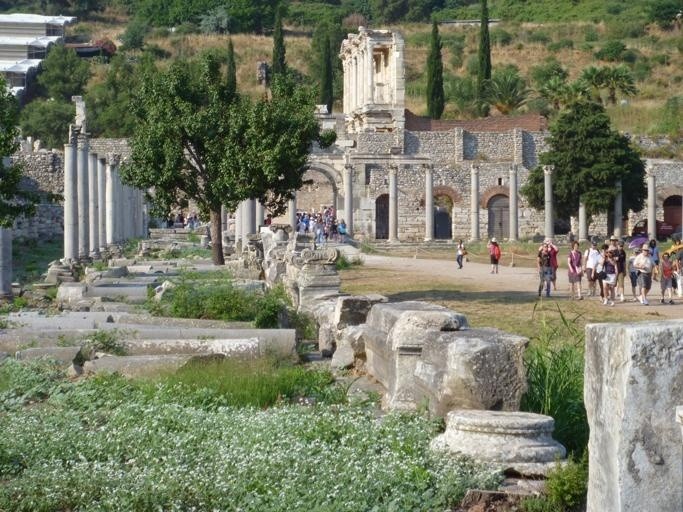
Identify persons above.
[264,214,272,225]
[487,238,500,273]
[296,204,347,243]
[567,235,683,306]
[537,237,559,297]
[456,239,464,269]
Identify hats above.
[591,236,652,250]
[491,237,496,242]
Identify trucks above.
[631,219,680,241]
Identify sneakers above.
[601,295,675,306]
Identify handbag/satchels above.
[575,266,583,276]
[597,272,606,278]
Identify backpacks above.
[494,245,501,260]
